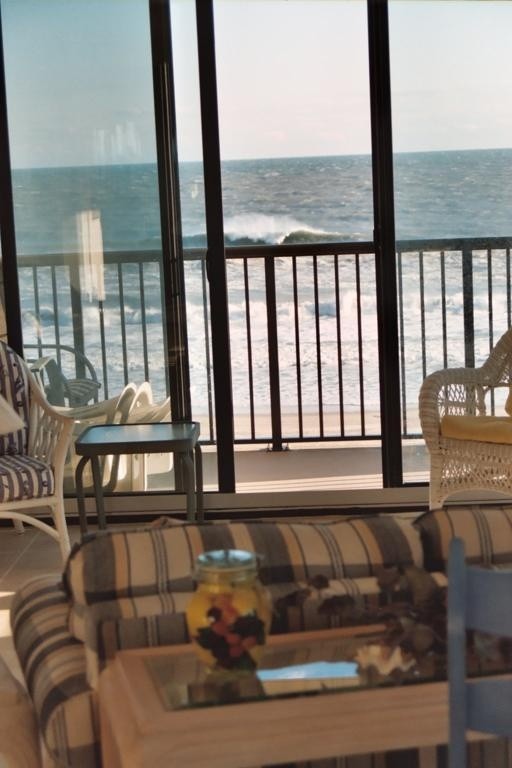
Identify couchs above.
[10,504,512,768]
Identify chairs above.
[419,329,512,512]
[0,341,76,563]
[23,344,101,408]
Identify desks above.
[75,420,202,536]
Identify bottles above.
[186,550,274,676]
